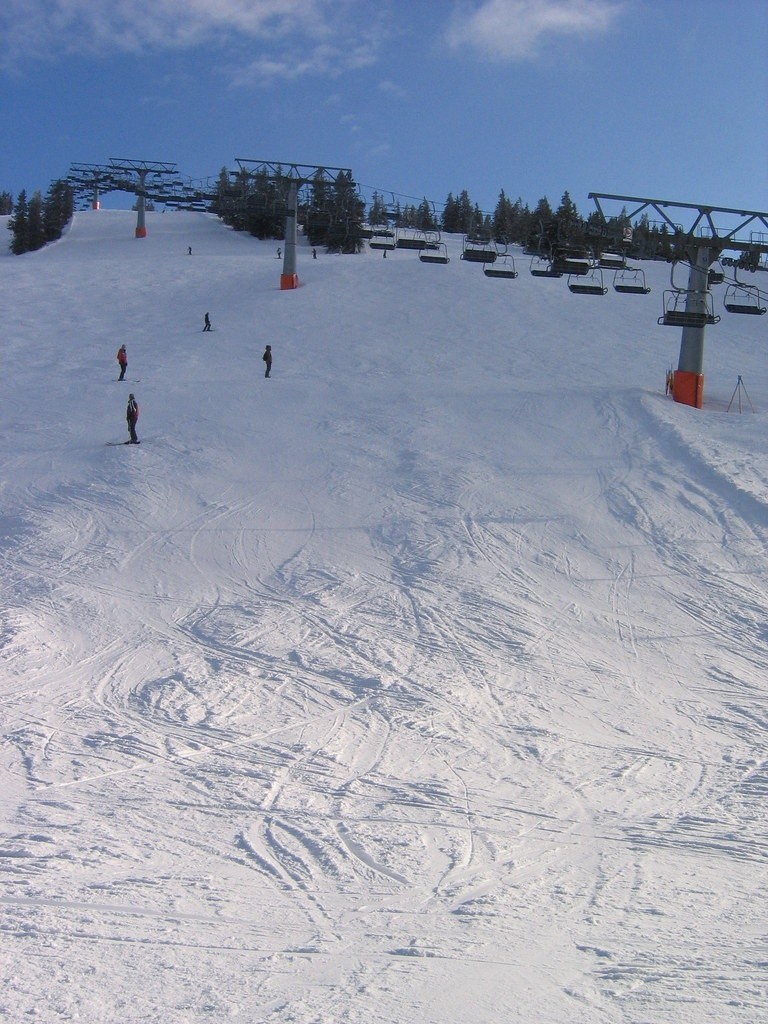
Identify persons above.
[126,394,139,444]
[117,345,128,381]
[188,247,192,255]
[202,312,211,332]
[312,248,317,259]
[265,345,272,378]
[707,269,715,289]
[277,248,281,258]
